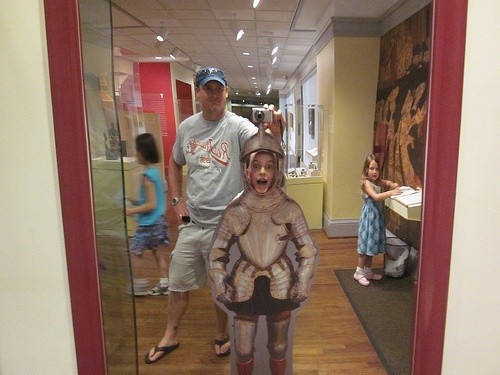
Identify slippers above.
[144,342,179,364]
[215,333,230,356]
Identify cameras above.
[252,107,272,123]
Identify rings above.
[179,213,182,216]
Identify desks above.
[384,186,422,221]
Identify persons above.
[144,67,286,367]
[123,132,172,295]
[353,154,403,286]
[246,150,275,194]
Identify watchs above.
[172,195,182,206]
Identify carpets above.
[334,268,414,375]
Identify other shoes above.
[365,272,382,281]
[353,272,369,286]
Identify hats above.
[196,68,227,88]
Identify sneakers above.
[147,284,169,296]
[126,286,152,295]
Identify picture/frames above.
[309,109,314,135]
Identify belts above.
[182,216,194,223]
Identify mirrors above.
[44,0,468,375]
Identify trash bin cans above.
[384,237,411,279]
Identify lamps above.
[268,39,278,55]
[267,51,277,64]
[156,27,169,41]
[233,14,244,40]
[170,48,179,59]
